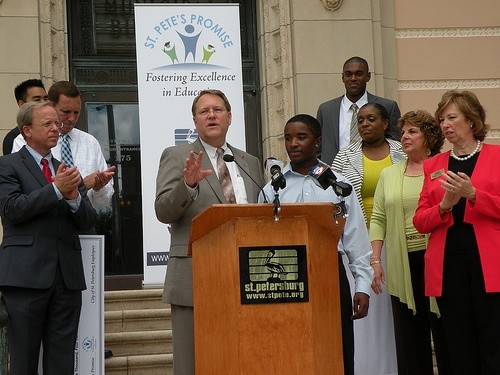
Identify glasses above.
[26,119,63,128]
[196,105,228,115]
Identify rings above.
[452,186,455,189]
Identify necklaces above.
[451,139,480,161]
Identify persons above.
[317,57,401,166]
[369,109,445,375]
[0,101,98,375]
[412,88,500,375]
[331,102,408,375]
[153,89,266,374]
[258,113,375,375]
[10,80,116,359]
[3,78,49,154]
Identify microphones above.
[309,163,352,197]
[223,154,267,203]
[270,165,286,207]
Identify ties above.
[216,147,236,204]
[41,159,53,185]
[60,133,75,168]
[350,104,361,145]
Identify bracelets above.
[370,256,381,260]
[370,261,381,266]
[439,202,453,212]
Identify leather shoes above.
[104,350,113,359]
